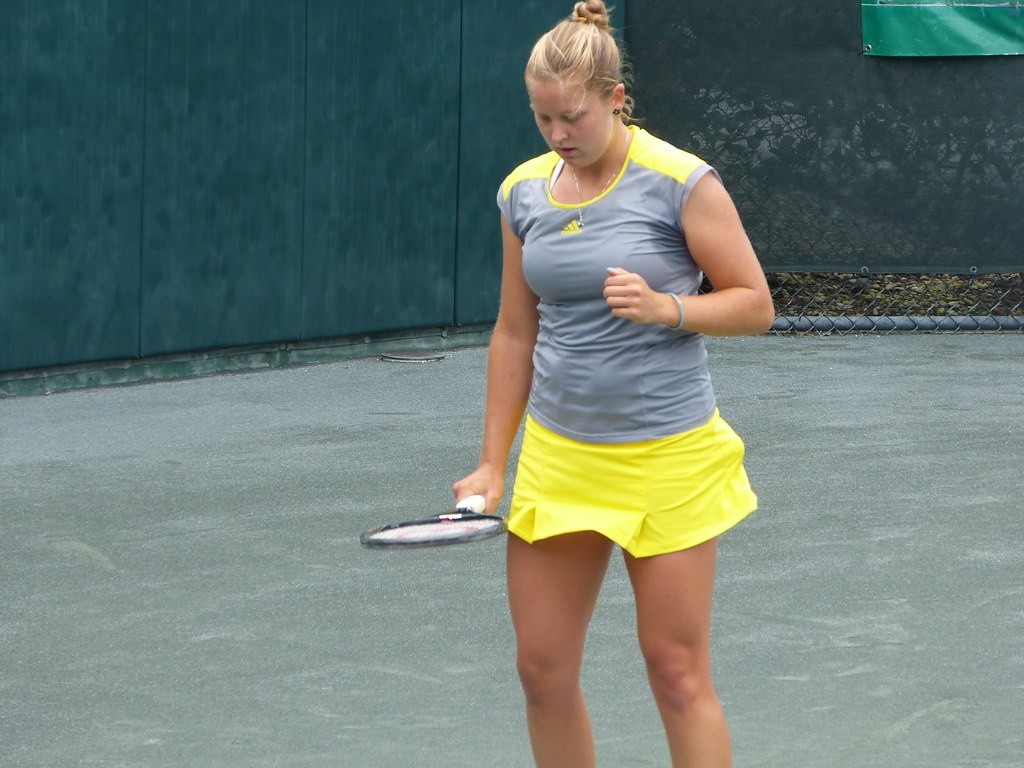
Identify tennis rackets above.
[359,494,508,550]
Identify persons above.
[452,0,775,768]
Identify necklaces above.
[569,126,635,228]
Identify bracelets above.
[665,291,685,334]
